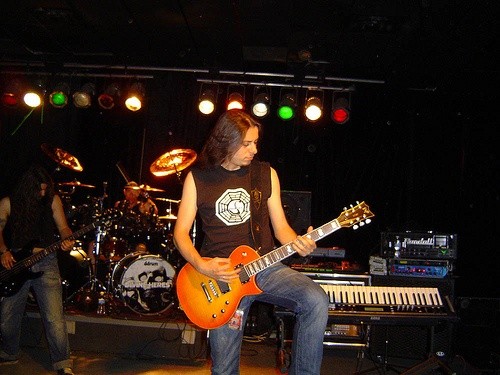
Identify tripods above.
[357,325,405,375]
[62,224,122,319]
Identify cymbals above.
[124,183,165,193]
[158,213,178,221]
[150,149,198,177]
[58,182,95,189]
[41,142,85,172]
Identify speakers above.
[369,275,458,362]
[280,190,312,235]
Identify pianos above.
[272,284,457,375]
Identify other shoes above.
[57,367,74,375]
[0,358,19,365]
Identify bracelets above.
[0,249,9,256]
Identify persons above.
[0,165,158,375]
[172,107,329,375]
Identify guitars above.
[0,209,125,297]
[174,201,375,332]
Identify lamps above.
[123,83,146,113]
[72,82,96,108]
[224,84,246,111]
[277,88,298,121]
[98,82,121,110]
[0,77,25,109]
[49,82,72,108]
[197,82,220,117]
[304,90,325,122]
[21,80,50,108]
[331,90,351,125]
[250,86,273,120]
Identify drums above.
[70,200,164,245]
[111,250,177,315]
